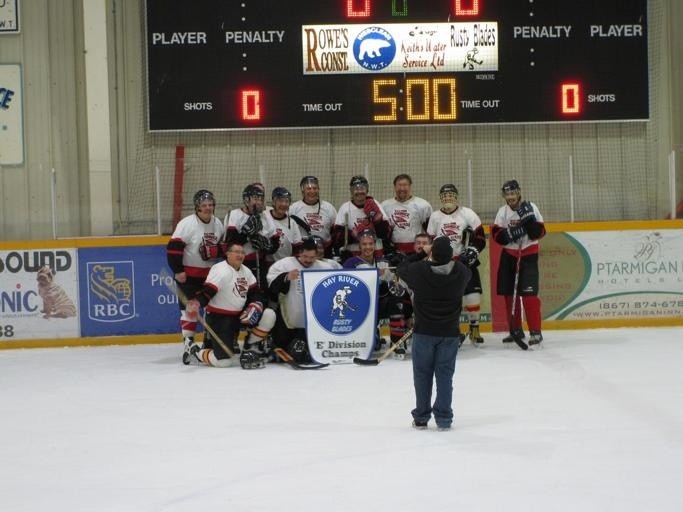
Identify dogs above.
[36,266,77,319]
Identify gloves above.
[517,200,536,232]
[240,301,264,327]
[185,299,200,320]
[504,223,525,243]
[460,248,479,269]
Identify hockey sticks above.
[509,238,528,350]
[266,338,330,370]
[156,269,240,364]
[291,214,313,240]
[353,326,412,366]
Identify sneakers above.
[230,340,407,369]
[528,334,543,345]
[501,330,523,342]
[468,325,484,343]
[437,421,450,430]
[183,342,199,364]
[411,421,427,430]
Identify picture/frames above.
[0,0,21,35]
[0,62,24,168]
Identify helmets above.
[349,177,366,186]
[503,180,519,192]
[193,189,214,204]
[243,183,263,196]
[300,176,317,189]
[440,184,457,204]
[272,188,289,199]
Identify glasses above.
[226,250,245,254]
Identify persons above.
[400,235,472,430]
[166,173,487,370]
[491,180,546,346]
